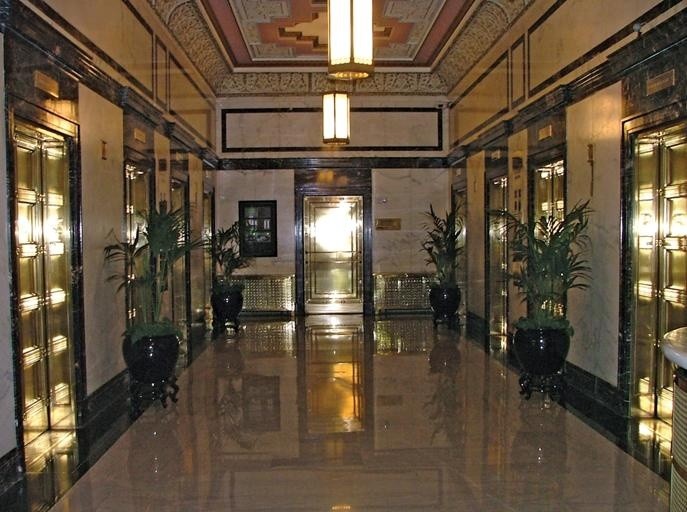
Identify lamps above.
[322,94,351,145]
[327,0,373,81]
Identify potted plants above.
[418,200,468,328]
[488,198,595,400]
[103,207,208,409]
[203,221,257,333]
